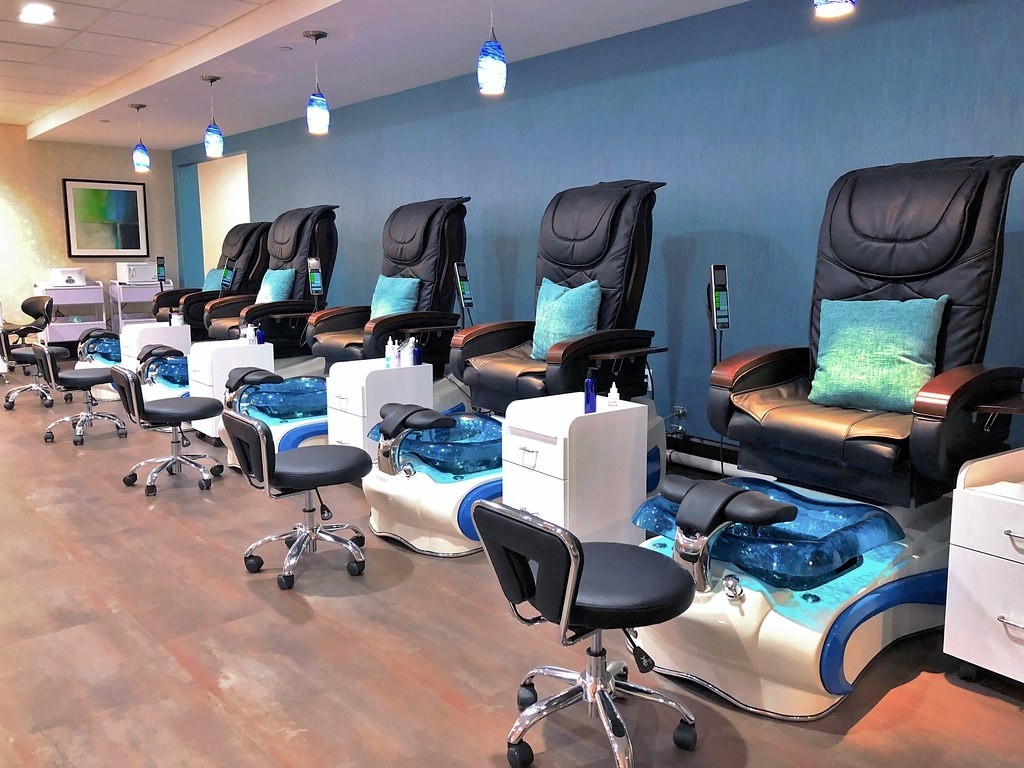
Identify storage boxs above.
[47,267,88,287]
[117,261,159,284]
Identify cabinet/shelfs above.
[502,391,647,546]
[33,280,107,347]
[326,357,433,464]
[108,278,174,334]
[188,339,276,447]
[943,446,1024,684]
[119,322,192,373]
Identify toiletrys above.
[583,366,600,414]
[167,305,185,326]
[607,382,621,409]
[385,336,422,369]
[238,321,265,346]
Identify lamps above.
[200,75,224,158]
[302,30,330,135]
[476,0,507,97]
[813,0,857,18]
[128,103,150,173]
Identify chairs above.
[0,154,1024,768]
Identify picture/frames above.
[61,178,150,258]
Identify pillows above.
[255,267,296,305]
[202,267,233,291]
[530,276,601,361]
[807,293,951,414]
[370,273,421,321]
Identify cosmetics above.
[168,306,184,326]
[607,381,619,410]
[239,322,264,345]
[384,336,421,368]
[584,367,600,414]
